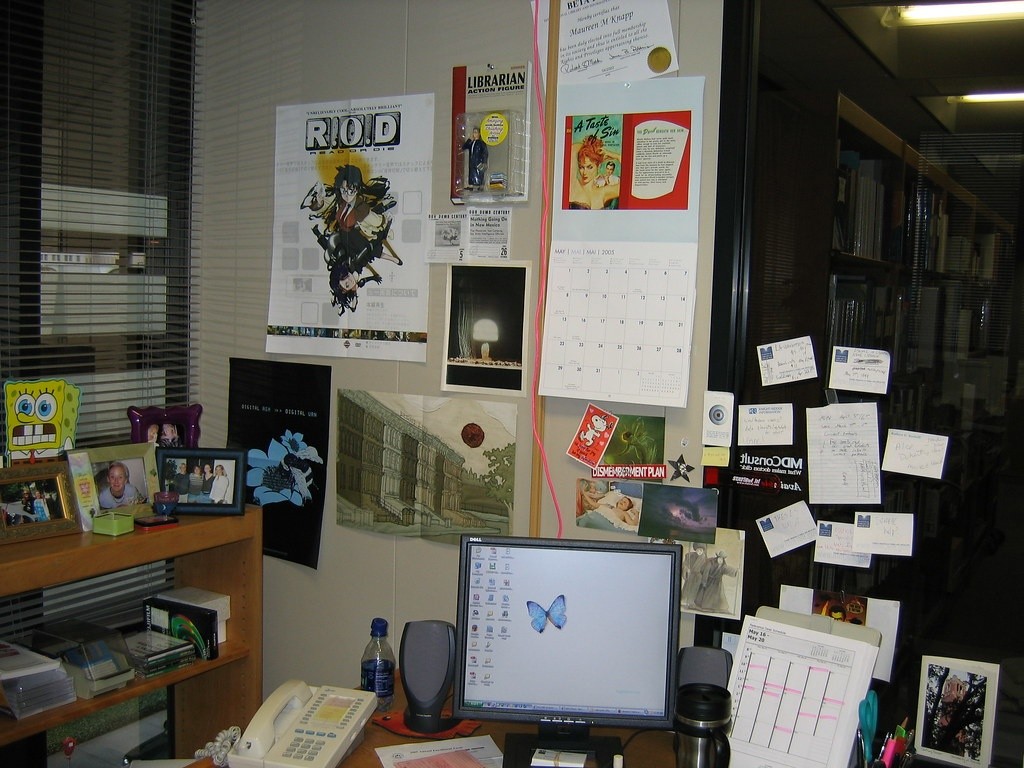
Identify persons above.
[148,424,182,448]
[462,127,489,187]
[21,490,62,523]
[99,462,142,509]
[173,463,229,503]
[579,488,639,527]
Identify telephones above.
[225,679,379,768]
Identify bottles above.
[360,618,396,710]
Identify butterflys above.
[525,594,567,635]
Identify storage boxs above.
[92,512,135,537]
[142,586,230,662]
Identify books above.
[0,640,60,680]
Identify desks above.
[184,664,678,768]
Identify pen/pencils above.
[856,717,917,768]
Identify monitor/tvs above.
[451,532,683,768]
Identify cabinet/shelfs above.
[1,501,264,760]
[830,90,1016,682]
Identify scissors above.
[859,691,878,768]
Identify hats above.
[693,542,706,551]
[715,551,728,559]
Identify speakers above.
[676,646,733,688]
[398,619,457,734]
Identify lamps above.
[473,319,499,362]
[879,0,1024,30]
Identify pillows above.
[598,492,642,512]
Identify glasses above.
[23,493,29,494]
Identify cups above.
[673,683,733,768]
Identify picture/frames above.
[127,403,203,449]
[155,447,248,516]
[66,443,160,532]
[0,461,83,548]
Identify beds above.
[576,503,641,535]
